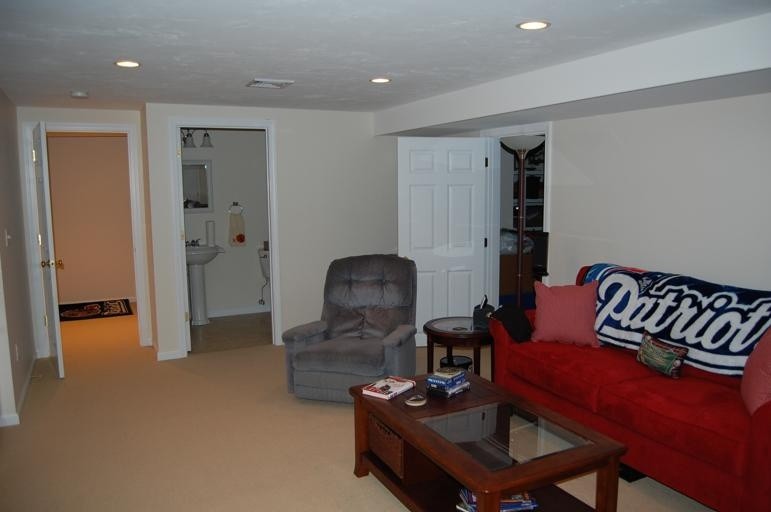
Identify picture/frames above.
[182,159,214,213]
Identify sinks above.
[186,244,224,264]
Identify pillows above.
[741,326,771,416]
[532,281,600,348]
[635,330,688,379]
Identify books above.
[426,381,473,399]
[361,375,417,399]
[428,378,467,391]
[426,367,467,387]
[456,484,540,512]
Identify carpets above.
[59,299,133,322]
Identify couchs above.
[488,263,771,512]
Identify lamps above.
[181,129,213,148]
[501,136,545,310]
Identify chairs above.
[282,254,417,404]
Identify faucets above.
[191,240,195,245]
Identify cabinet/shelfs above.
[501,147,544,232]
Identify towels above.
[230,213,246,247]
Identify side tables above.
[423,317,492,376]
